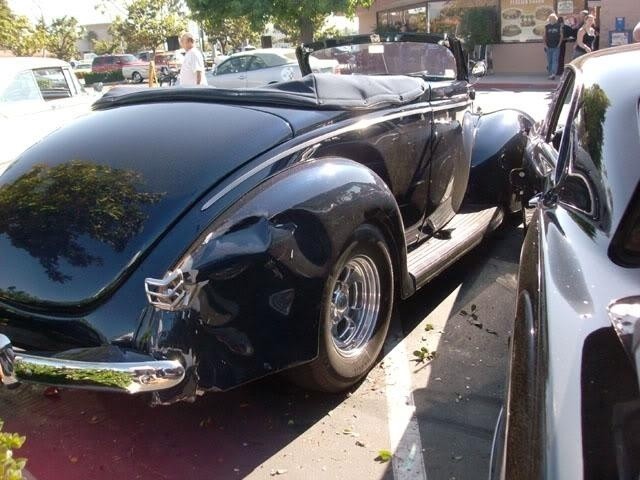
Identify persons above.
[542,9,597,80]
[179,32,208,86]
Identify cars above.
[67,49,189,85]
[203,47,341,89]
[0,28,543,412]
[0,56,107,178]
[484,35,640,480]
